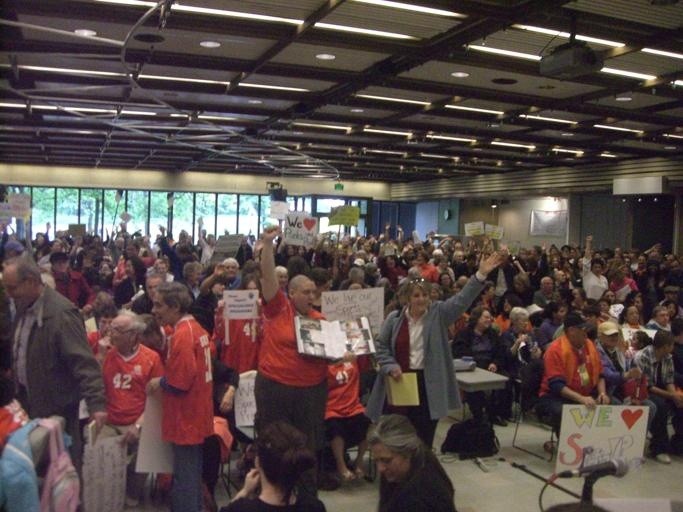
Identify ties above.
[11,316,25,393]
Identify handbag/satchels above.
[440,416,502,461]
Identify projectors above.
[539,47,604,80]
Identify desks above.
[447,366,509,423]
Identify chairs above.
[510,358,557,463]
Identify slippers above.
[335,469,358,483]
[368,470,376,481]
[349,462,364,480]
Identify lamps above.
[490,199,497,209]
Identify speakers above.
[272,189,287,202]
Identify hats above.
[354,258,366,266]
[49,252,69,263]
[664,286,681,293]
[598,321,618,336]
[526,304,545,318]
[5,240,25,253]
[564,310,594,328]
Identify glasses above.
[408,278,425,285]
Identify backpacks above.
[37,417,81,512]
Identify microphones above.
[557,457,628,478]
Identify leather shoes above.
[489,414,507,427]
[656,453,672,464]
